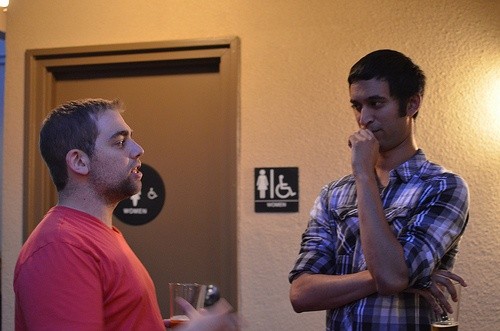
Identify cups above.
[169,282,207,320]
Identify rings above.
[424,281,434,289]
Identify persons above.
[10,97,233,331]
[287,47,471,330]
